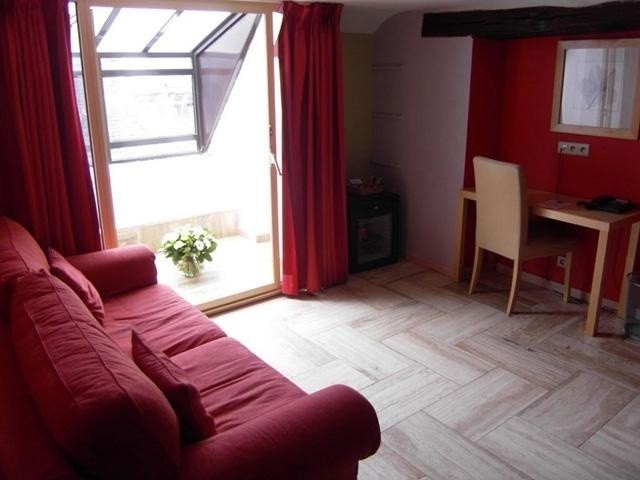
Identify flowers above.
[157,223,217,271]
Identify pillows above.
[127,327,217,445]
[46,248,105,326]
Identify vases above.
[177,259,206,278]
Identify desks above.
[451,188,640,338]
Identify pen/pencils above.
[362,175,384,185]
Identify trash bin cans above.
[625,271,640,342]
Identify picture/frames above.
[548,38,640,141]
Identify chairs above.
[468,155,583,317]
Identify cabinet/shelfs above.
[345,188,407,276]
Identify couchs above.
[1,215,380,480]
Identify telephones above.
[589,195,637,213]
